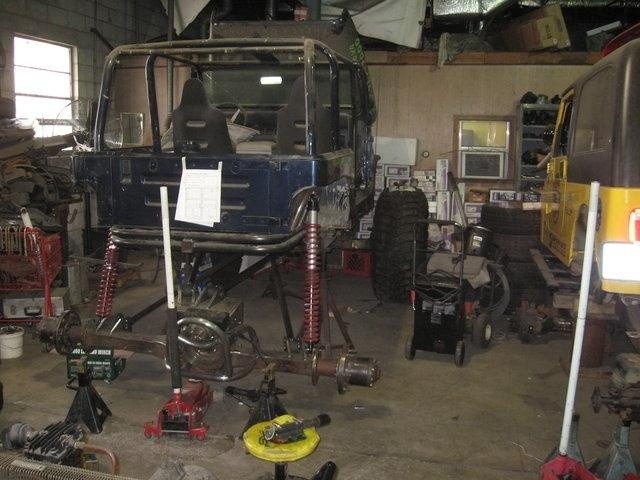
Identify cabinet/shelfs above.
[514,102,560,192]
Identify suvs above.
[35,31,429,396]
[522,33,640,331]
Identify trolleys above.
[406,212,499,368]
[0,222,65,356]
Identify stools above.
[243,414,321,480]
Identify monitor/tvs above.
[458,121,511,151]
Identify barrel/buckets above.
[0,326,25,359]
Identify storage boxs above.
[358,158,542,241]
[491,5,574,52]
